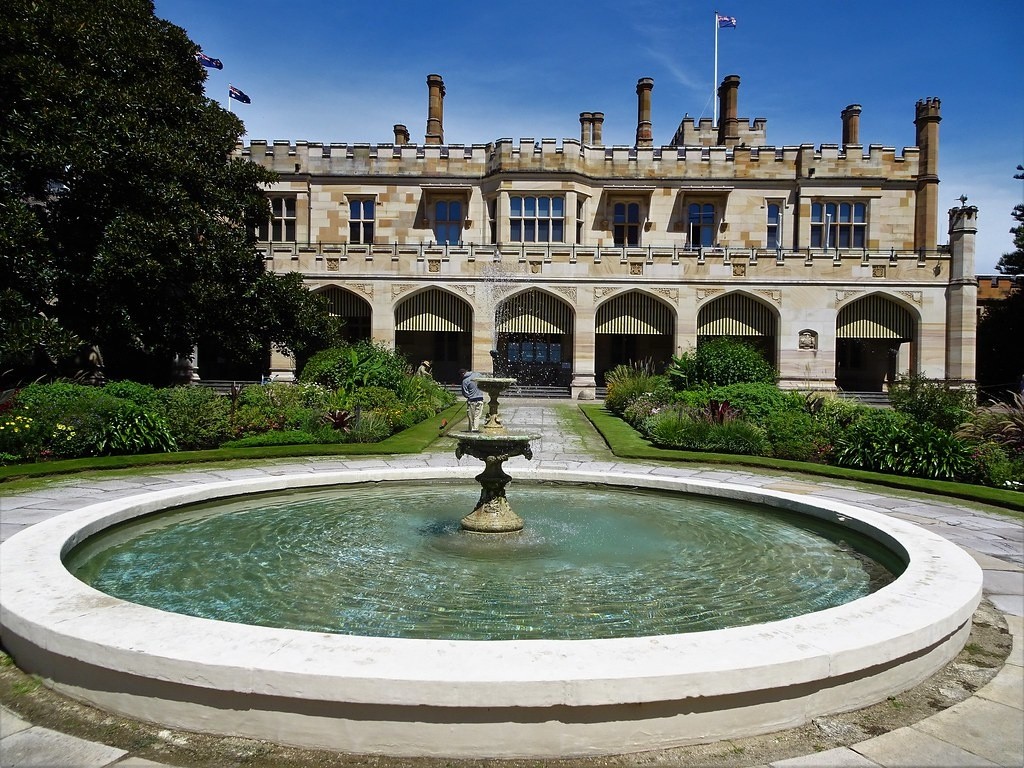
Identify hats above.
[421,361,430,367]
[458,369,466,380]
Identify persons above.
[416,361,431,379]
[459,368,485,432]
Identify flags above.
[718,15,737,30]
